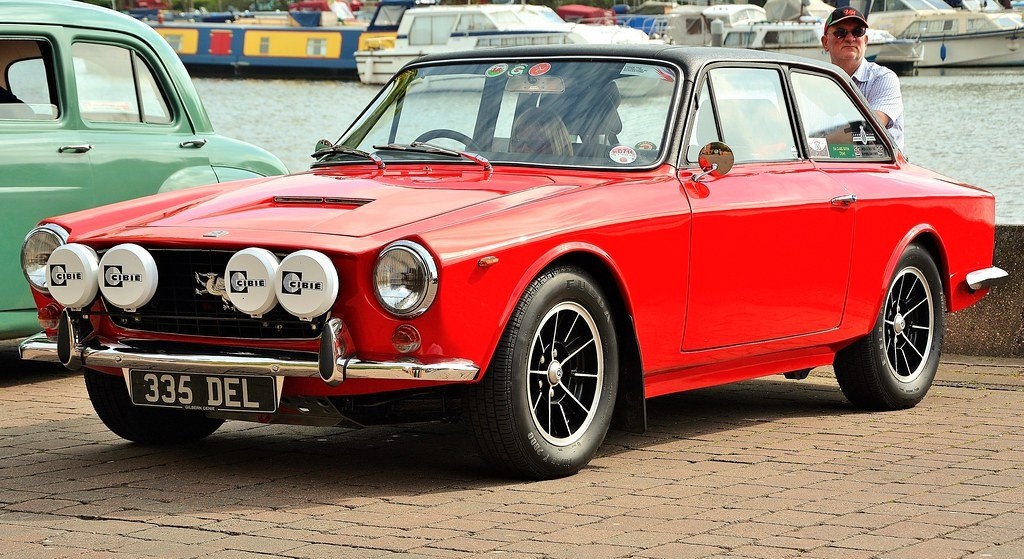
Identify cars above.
[0,0,291,351]
[15,46,1011,477]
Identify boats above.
[353,0,1024,98]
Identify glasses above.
[828,26,867,39]
[510,138,551,153]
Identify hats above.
[824,6,869,34]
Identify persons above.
[509,107,574,156]
[808,7,904,158]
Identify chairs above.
[559,88,622,157]
[689,96,789,161]
